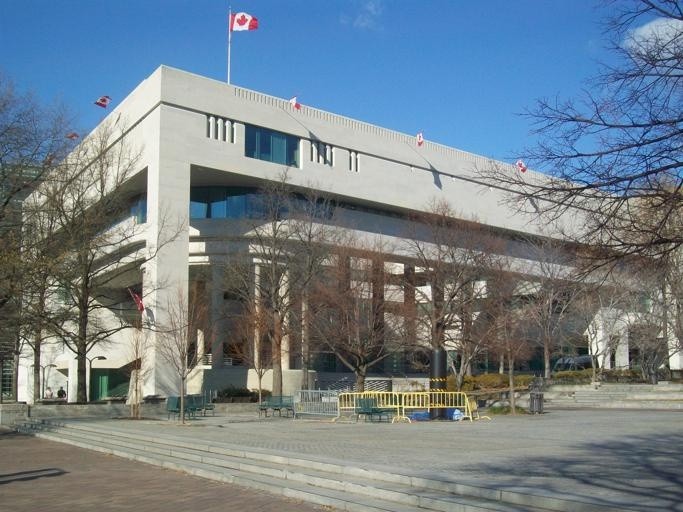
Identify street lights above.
[31,364,57,399]
[75,355,106,402]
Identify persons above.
[57,387,66,398]
[44,387,53,399]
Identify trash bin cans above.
[530,393,543,414]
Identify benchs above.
[167,394,215,421]
[353,398,396,423]
[256,395,294,418]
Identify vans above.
[552,356,599,372]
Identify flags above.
[128,288,144,313]
[64,133,80,139]
[516,160,527,173]
[416,131,424,146]
[230,12,258,31]
[290,97,301,109]
[95,96,112,108]
